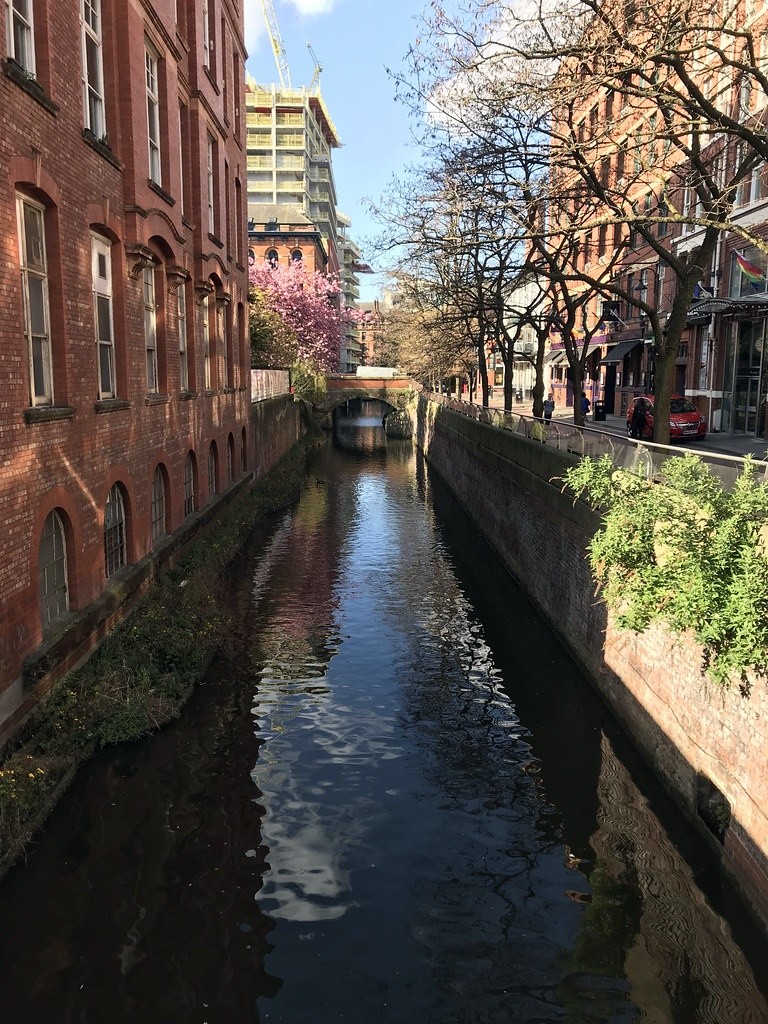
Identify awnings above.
[549,346,600,370]
[597,339,643,365]
[542,349,563,364]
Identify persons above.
[632,396,647,438]
[543,392,554,426]
[580,393,591,415]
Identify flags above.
[733,250,766,291]
[693,284,712,301]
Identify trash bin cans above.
[515,390,523,404]
[488,385,491,395]
[592,400,607,421]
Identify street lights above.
[633,265,660,396]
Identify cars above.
[625,393,707,444]
